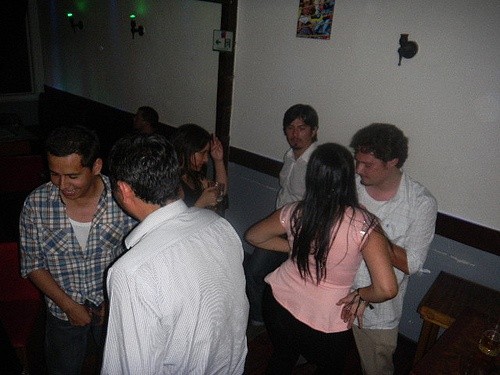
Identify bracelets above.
[344,288,369,318]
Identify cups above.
[207,176,225,202]
[478,325,500,356]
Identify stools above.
[412,270,500,370]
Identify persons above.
[244,143,399,375]
[132,106,159,135]
[19,122,140,375]
[169,123,229,218]
[100,132,251,375]
[247,104,321,327]
[350,123,437,375]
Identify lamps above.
[67,13,83,34]
[397,34,418,66]
[130,15,144,39]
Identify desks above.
[410,309,500,375]
